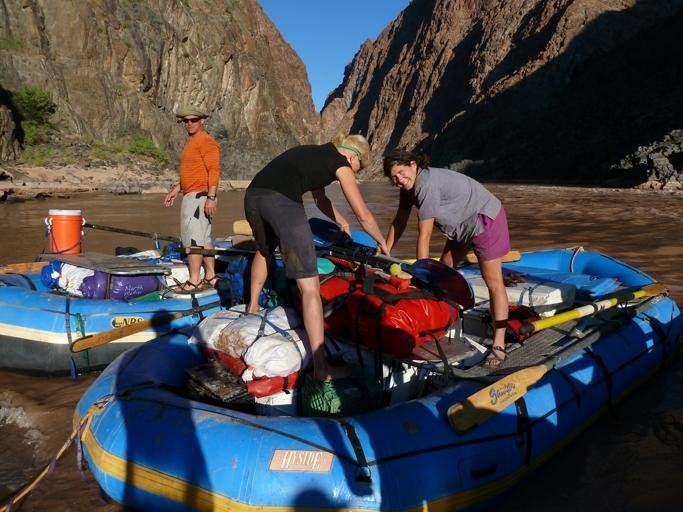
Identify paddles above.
[519,282,670,334]
[447,292,666,434]
[309,218,475,309]
[70,302,220,351]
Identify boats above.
[0,225,378,384]
[68,237,681,512]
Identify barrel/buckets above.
[45,208,83,255]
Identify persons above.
[244,133,391,381]
[383,149,510,367]
[163,105,221,293]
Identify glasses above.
[182,116,199,124]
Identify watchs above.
[206,194,217,200]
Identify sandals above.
[175,276,216,293]
[485,347,508,367]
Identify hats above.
[175,105,208,118]
